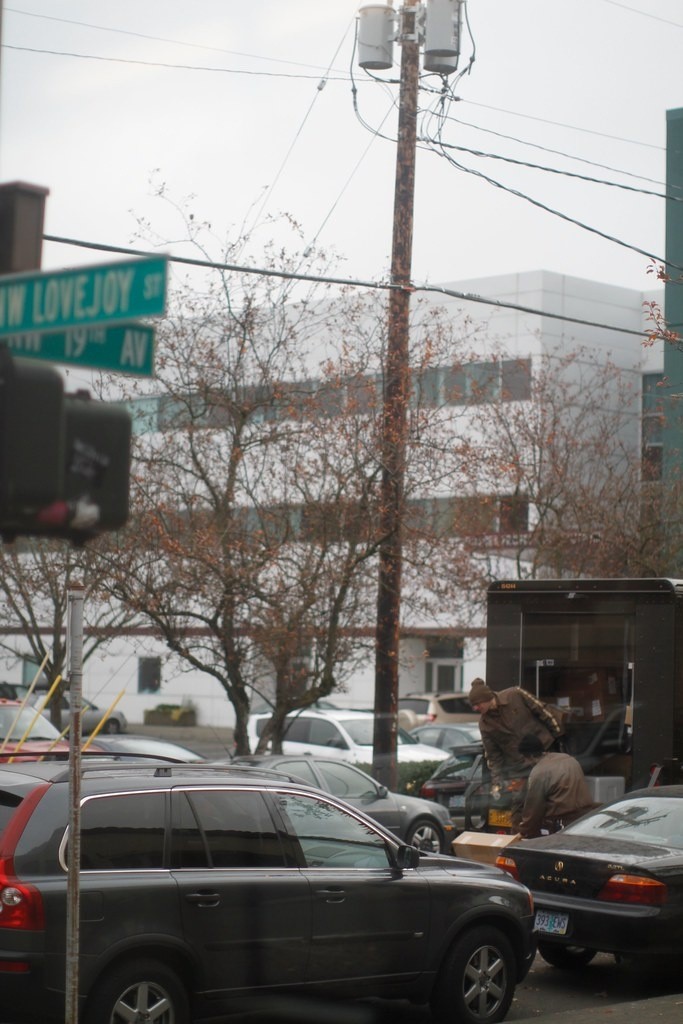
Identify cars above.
[203,755,456,857]
[244,709,455,798]
[409,723,527,818]
[0,696,104,766]
[466,726,629,837]
[396,691,483,728]
[0,683,127,739]
[494,786,682,983]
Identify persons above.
[518,735,593,838]
[468,677,567,835]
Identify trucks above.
[485,577,683,795]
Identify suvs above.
[0,760,534,1023]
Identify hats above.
[468,677,494,705]
